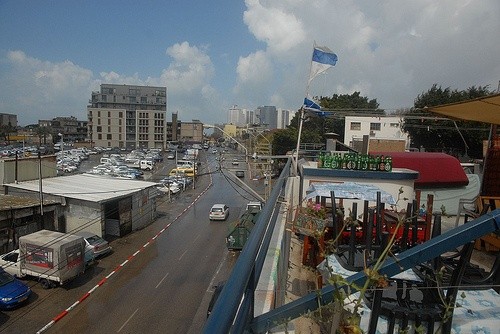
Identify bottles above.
[318,152,393,172]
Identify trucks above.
[0,230,85,289]
[340,208,427,246]
[225,201,262,251]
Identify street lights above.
[201,123,272,199]
[57,133,64,172]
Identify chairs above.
[456,192,500,251]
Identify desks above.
[302,161,419,220]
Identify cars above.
[0,141,244,196]
[209,203,229,221]
[0,266,33,312]
[206,280,227,318]
[72,232,113,266]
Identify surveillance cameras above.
[251,153,257,161]
